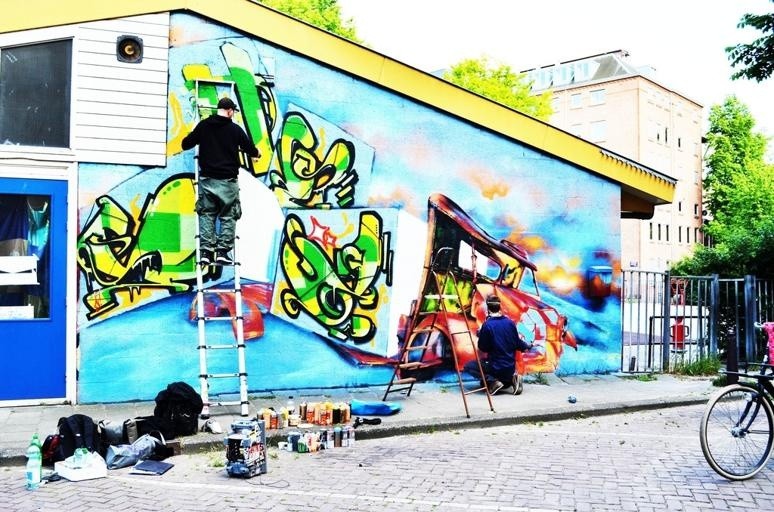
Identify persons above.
[179,97,260,265]
[463,294,528,396]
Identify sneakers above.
[489,380,504,396]
[202,252,233,263]
[513,375,523,395]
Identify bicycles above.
[700,321,774,481]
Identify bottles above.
[288,425,356,454]
[26,432,43,492]
[288,395,352,426]
[257,407,288,429]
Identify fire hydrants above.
[670,317,689,354]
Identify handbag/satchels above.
[153,382,203,439]
[42,414,153,466]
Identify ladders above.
[194,78,249,420]
[382,247,493,418]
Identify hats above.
[486,295,501,305]
[218,97,237,109]
